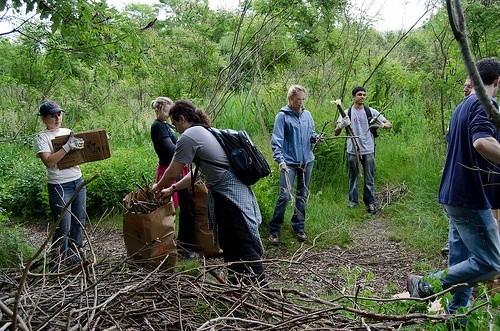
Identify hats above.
[36,102,65,116]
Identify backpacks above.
[187,124,271,186]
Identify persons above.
[268,86,324,245]
[335,87,392,214]
[35,102,109,271]
[150,96,190,209]
[407,58,500,328]
[149,100,269,288]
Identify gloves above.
[338,115,351,129]
[278,162,290,173]
[62,131,84,153]
[370,117,385,129]
[316,135,324,146]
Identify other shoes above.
[368,203,376,214]
[349,201,358,207]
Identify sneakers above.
[268,234,279,242]
[407,274,427,306]
[296,232,307,241]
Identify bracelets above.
[172,184,178,192]
